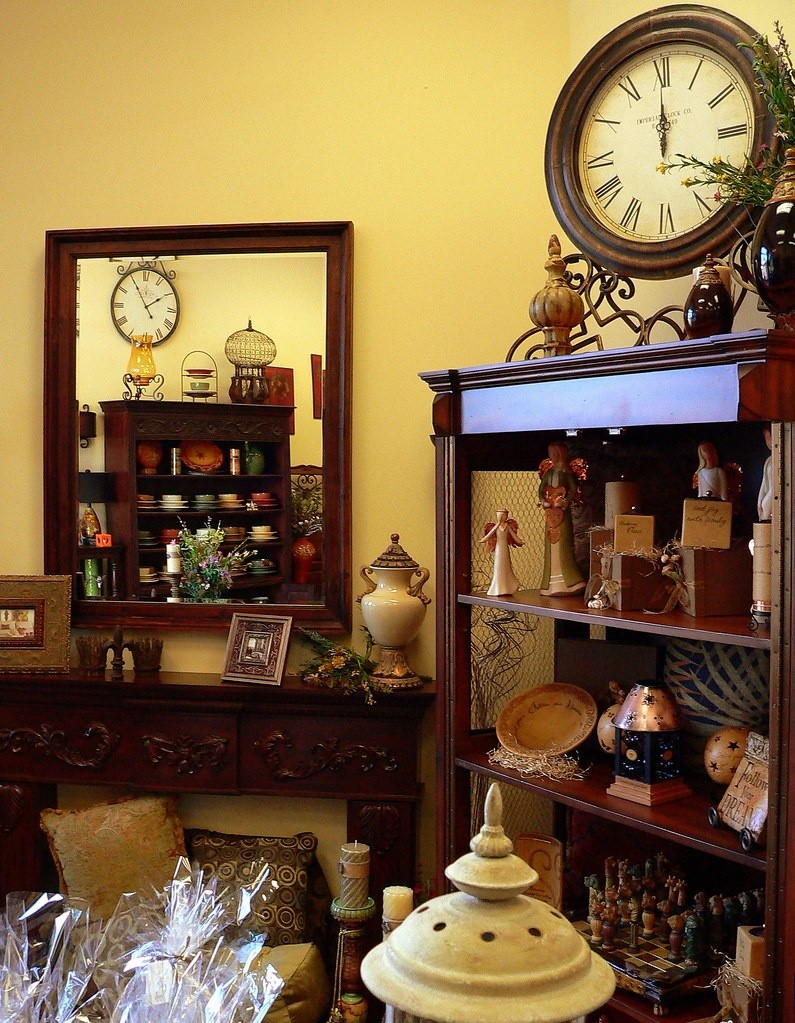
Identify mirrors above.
[43,220,354,637]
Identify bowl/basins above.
[163,566,167,572]
[138,531,154,538]
[195,494,215,501]
[136,495,155,501]
[252,526,271,532]
[140,568,151,575]
[251,559,269,567]
[160,530,181,536]
[162,495,182,501]
[196,529,215,536]
[218,494,238,500]
[222,527,245,534]
[255,493,272,499]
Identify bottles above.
[230,449,240,476]
[171,448,182,475]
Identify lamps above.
[78,469,115,545]
[122,335,164,402]
[80,404,97,449]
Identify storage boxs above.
[717,975,763,1023]
[606,554,675,612]
[677,549,753,618]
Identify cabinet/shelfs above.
[419,332,795,1023]
[98,400,292,602]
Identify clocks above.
[110,262,180,346]
[506,3,791,363]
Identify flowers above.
[292,485,323,537]
[170,516,258,603]
[656,20,795,209]
[298,625,432,706]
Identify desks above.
[1,668,436,943]
[78,541,126,601]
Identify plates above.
[230,565,279,579]
[138,532,278,549]
[496,683,598,758]
[140,572,184,586]
[137,499,280,512]
[179,441,223,472]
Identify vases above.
[292,537,315,584]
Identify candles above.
[341,840,369,909]
[166,544,180,573]
[383,885,413,921]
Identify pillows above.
[186,828,318,949]
[258,943,332,1023]
[40,792,191,927]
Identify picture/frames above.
[220,612,293,686]
[311,354,322,419]
[262,366,295,435]
[0,574,72,674]
